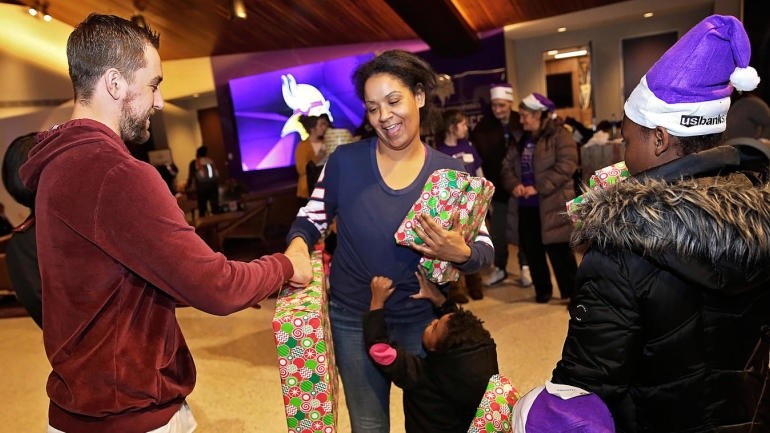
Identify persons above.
[283,50,499,433]
[550,14,770,433]
[185,113,335,218]
[435,82,622,304]
[0,14,314,433]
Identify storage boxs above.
[272,250,339,433]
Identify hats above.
[521,92,558,120]
[623,14,761,138]
[489,83,514,101]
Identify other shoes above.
[536,292,553,303]
[482,269,508,286]
[520,265,533,288]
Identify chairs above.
[218,196,272,255]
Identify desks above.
[196,210,245,251]
